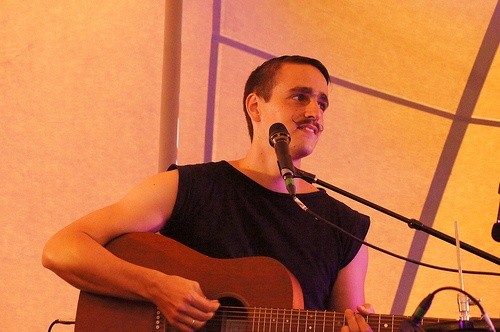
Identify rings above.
[191,319,194,327]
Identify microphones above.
[269,122,295,195]
[399,292,434,332]
[492,202,500,243]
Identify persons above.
[42,55,375,332]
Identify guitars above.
[75,231,500,332]
